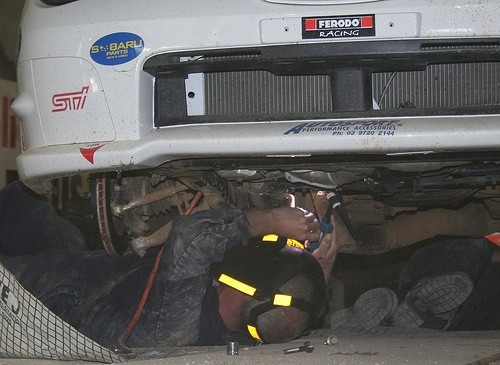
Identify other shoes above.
[392,269,473,328]
[329,286,397,332]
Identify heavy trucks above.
[7,0,500,259]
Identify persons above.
[1,179,340,359]
[319,234,494,334]
